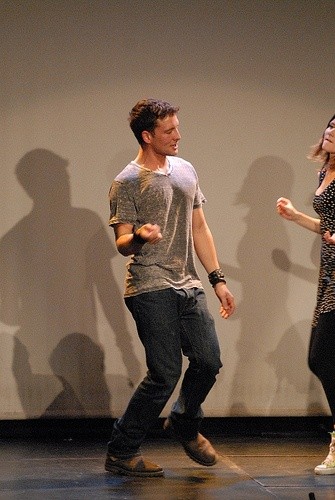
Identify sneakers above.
[313,430,335,475]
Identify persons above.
[276,114,335,475]
[104,98,235,477]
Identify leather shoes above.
[164,419,218,466]
[105,453,165,477]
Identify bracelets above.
[208,269,224,284]
[212,278,226,288]
